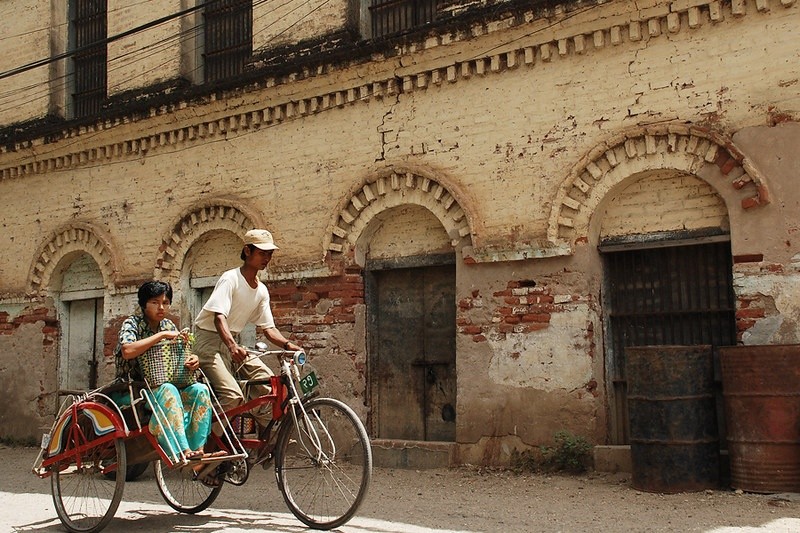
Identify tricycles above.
[30,339,373,533]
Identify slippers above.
[261,440,298,470]
[201,469,220,488]
[205,451,228,457]
[178,451,203,460]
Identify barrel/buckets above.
[717,343,800,493]
[624,344,720,494]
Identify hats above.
[244,229,279,251]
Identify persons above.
[115,280,228,481]
[192,229,306,488]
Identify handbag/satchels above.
[138,329,197,388]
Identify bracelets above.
[285,341,289,350]
